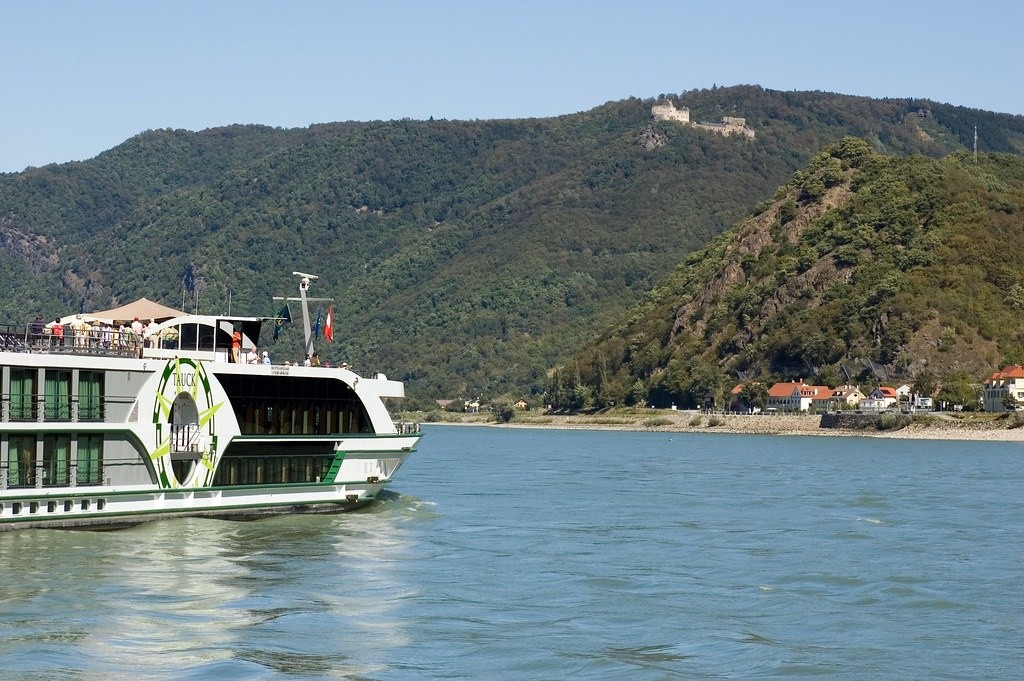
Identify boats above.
[0,261,422,533]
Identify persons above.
[82,316,179,359]
[51,317,64,352]
[231,327,241,364]
[69,313,84,353]
[303,354,311,367]
[30,315,46,345]
[262,351,271,365]
[148,318,159,360]
[247,346,259,364]
[310,352,320,367]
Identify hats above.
[262,350,269,357]
[76,314,82,319]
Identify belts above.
[151,333,157,335]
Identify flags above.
[272,325,283,343]
[315,305,321,336]
[273,305,291,327]
[323,304,333,343]
